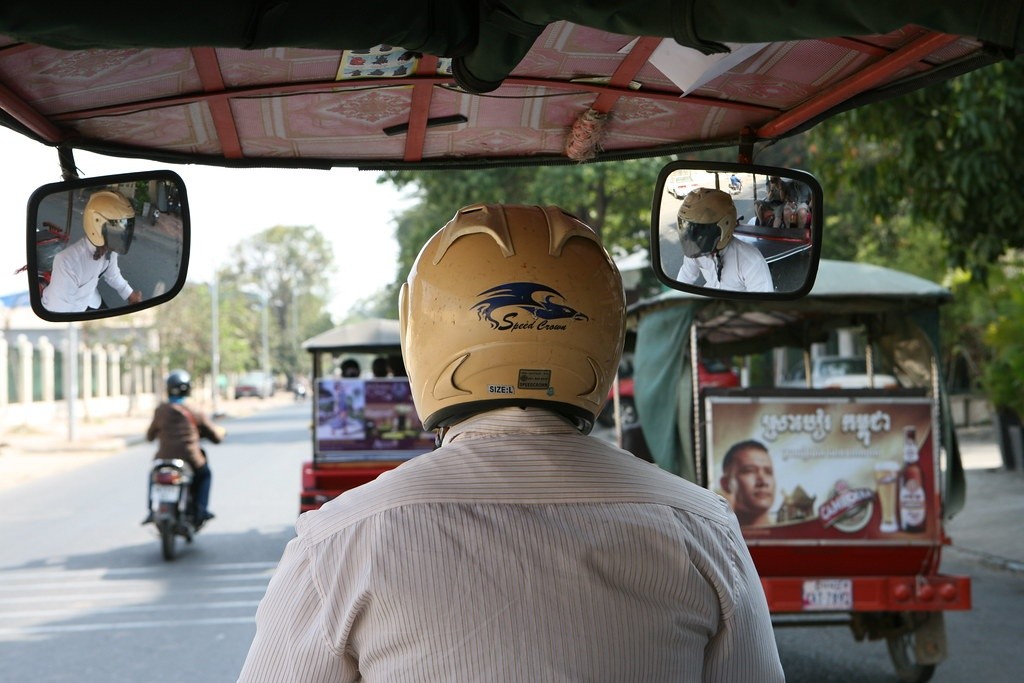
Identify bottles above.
[895,426,928,533]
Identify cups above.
[872,461,900,532]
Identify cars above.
[234,371,274,402]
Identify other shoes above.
[141,512,155,524]
[195,507,215,522]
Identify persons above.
[729,174,741,190]
[39,188,144,312]
[336,351,406,377]
[676,187,776,295]
[234,199,792,682]
[717,439,779,526]
[751,175,814,229]
[140,370,229,526]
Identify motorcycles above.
[602,244,973,679]
[299,318,445,524]
[140,424,223,564]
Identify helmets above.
[677,187,737,258]
[83,189,134,255]
[167,368,191,399]
[397,205,627,437]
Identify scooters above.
[291,383,308,401]
